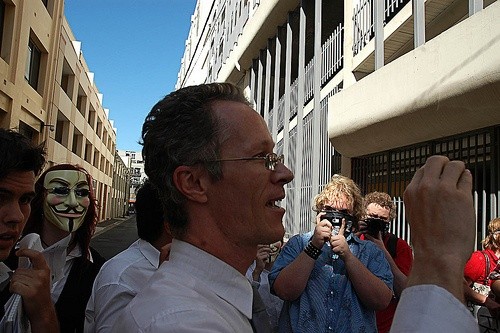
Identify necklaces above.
[41,239,50,248]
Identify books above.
[0,233,72,333]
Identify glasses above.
[190,153,284,170]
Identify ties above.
[251,279,277,333]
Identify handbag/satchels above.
[467,281,500,333]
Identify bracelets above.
[304,241,323,260]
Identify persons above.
[353,191,413,333]
[267,174,394,333]
[83,180,172,333]
[0,164,108,333]
[245,238,286,333]
[0,126,59,333]
[463,217,500,333]
[108,83,480,333]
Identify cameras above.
[363,216,390,238]
[321,213,352,233]
